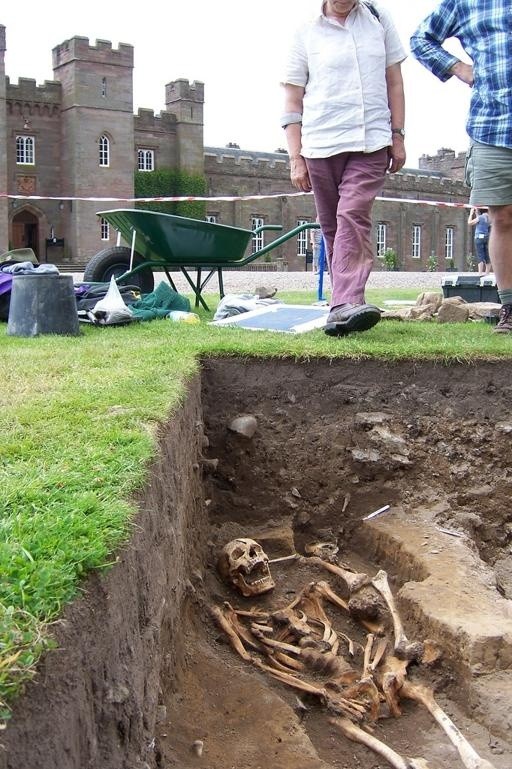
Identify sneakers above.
[494,303,511,334]
[323,302,382,336]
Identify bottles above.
[165,309,201,326]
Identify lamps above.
[58,200,72,213]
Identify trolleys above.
[81,207,323,313]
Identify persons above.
[467,206,491,278]
[278,0,407,338]
[408,2,510,335]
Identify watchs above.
[392,128,405,136]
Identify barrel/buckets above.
[7,274,80,336]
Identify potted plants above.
[447,260,457,272]
[387,249,401,272]
